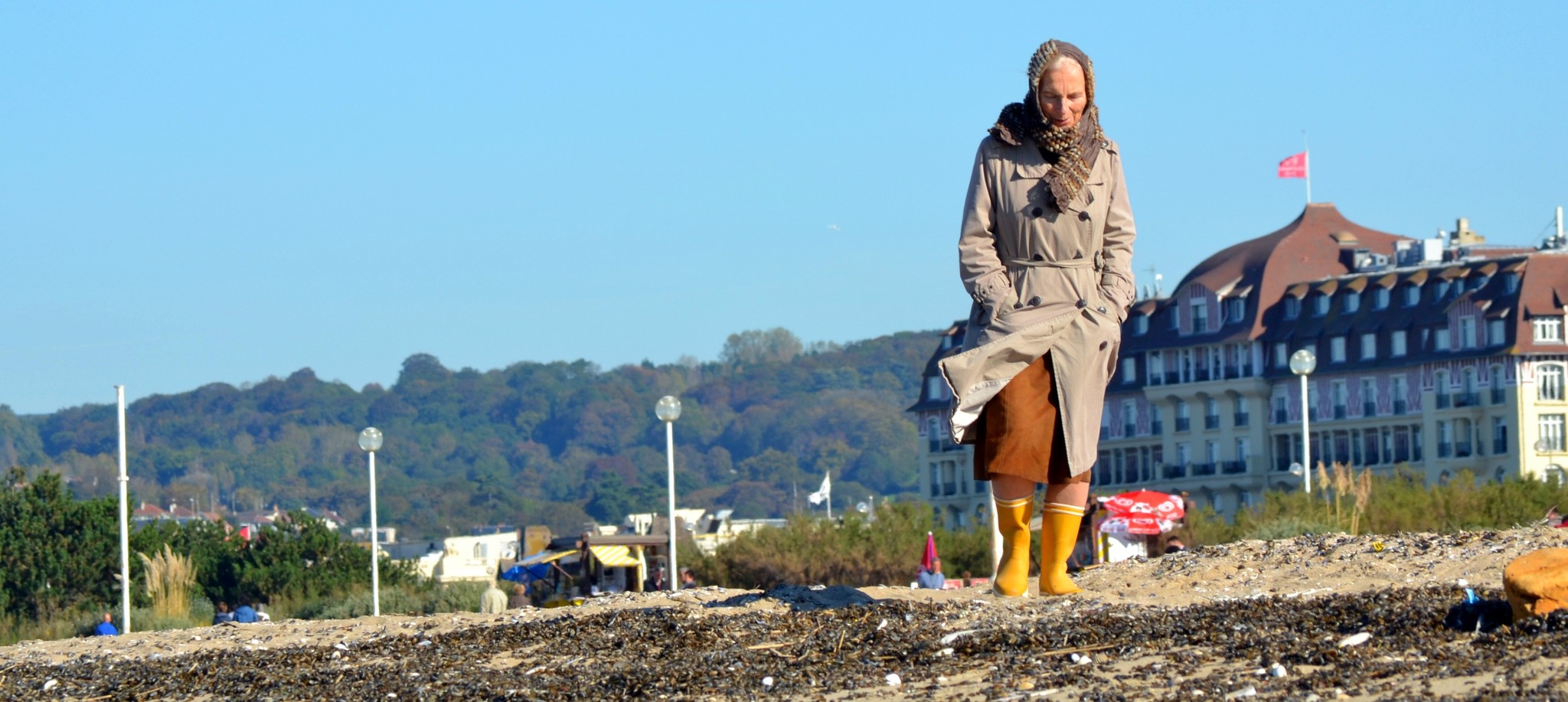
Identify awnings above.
[514,550,581,588]
[589,544,640,567]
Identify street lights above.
[358,426,384,617]
[1290,350,1316,492]
[655,395,683,591]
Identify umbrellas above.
[1098,487,1185,556]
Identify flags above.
[1278,151,1307,178]
[809,475,830,506]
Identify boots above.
[1038,500,1086,595]
[993,494,1034,599]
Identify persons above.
[679,566,696,589]
[212,593,270,626]
[510,584,532,609]
[919,557,945,589]
[93,612,119,636]
[1545,504,1568,528]
[480,577,509,614]
[1165,535,1186,554]
[936,38,1138,597]
[644,566,666,592]
